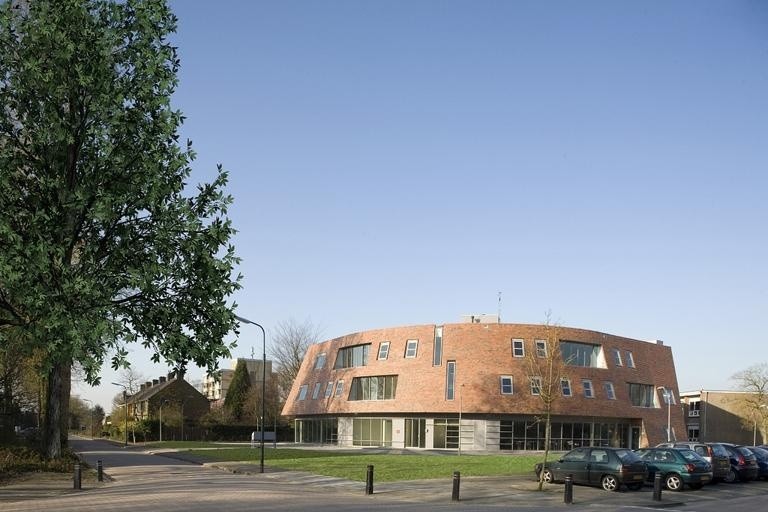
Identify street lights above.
[110,382,128,445]
[82,398,94,438]
[753,404,766,447]
[159,399,167,441]
[234,315,266,472]
[181,395,195,441]
[657,387,672,442]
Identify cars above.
[534,442,768,491]
